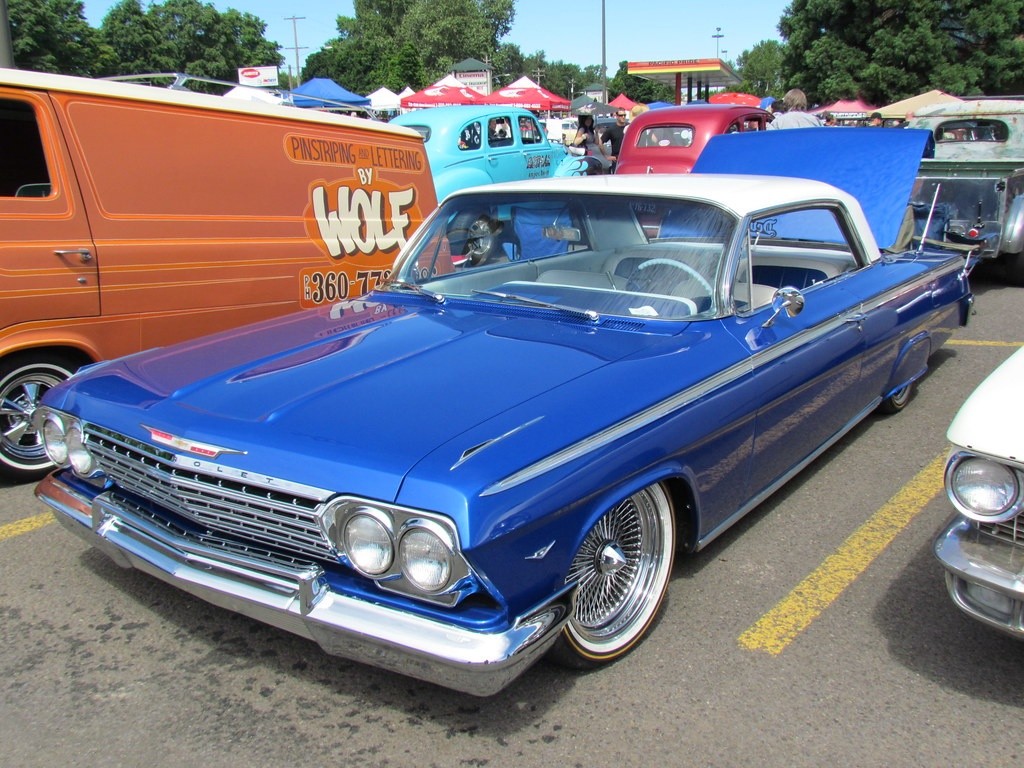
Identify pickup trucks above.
[909,99,1024,287]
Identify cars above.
[930,341,1024,645]
[615,106,776,175]
[31,127,981,700]
[389,106,604,207]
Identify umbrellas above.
[761,97,776,111]
[707,92,761,108]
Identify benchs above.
[601,250,840,295]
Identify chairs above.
[672,280,778,314]
[537,270,641,293]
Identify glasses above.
[617,114,626,117]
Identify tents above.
[219,71,709,115]
[805,89,967,120]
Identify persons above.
[622,105,649,136]
[830,118,837,126]
[573,115,613,175]
[598,107,630,174]
[765,88,824,130]
[824,114,834,126]
[747,120,759,131]
[488,118,498,139]
[869,113,882,126]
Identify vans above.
[1,65,457,486]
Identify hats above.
[629,105,649,123]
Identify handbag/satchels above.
[569,126,588,156]
